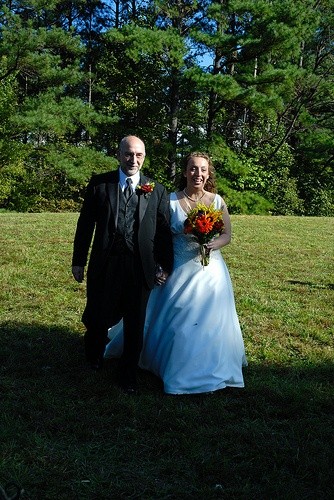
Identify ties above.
[124,178,133,200]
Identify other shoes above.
[89,359,101,370]
[123,384,138,394]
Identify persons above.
[104,151,247,396]
[72,136,176,395]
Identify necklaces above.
[183,190,206,202]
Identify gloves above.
[72,266,84,282]
[154,266,169,286]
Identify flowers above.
[137,182,155,199]
[183,199,227,271]
